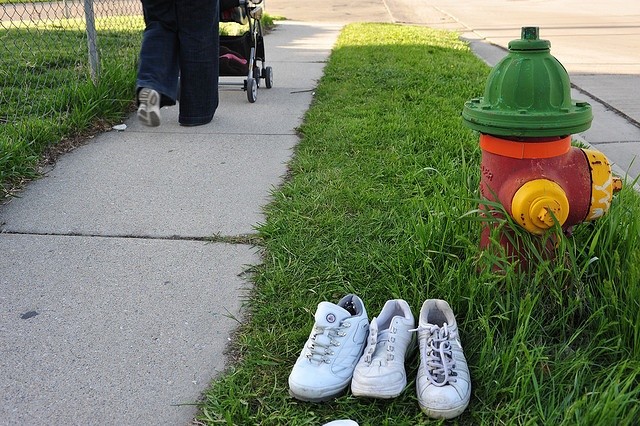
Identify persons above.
[133,1,220,127]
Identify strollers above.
[218,2,274,103]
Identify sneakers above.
[287,293,370,402]
[136,86,161,127]
[351,297,415,399]
[415,298,472,419]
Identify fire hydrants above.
[461,26,622,277]
